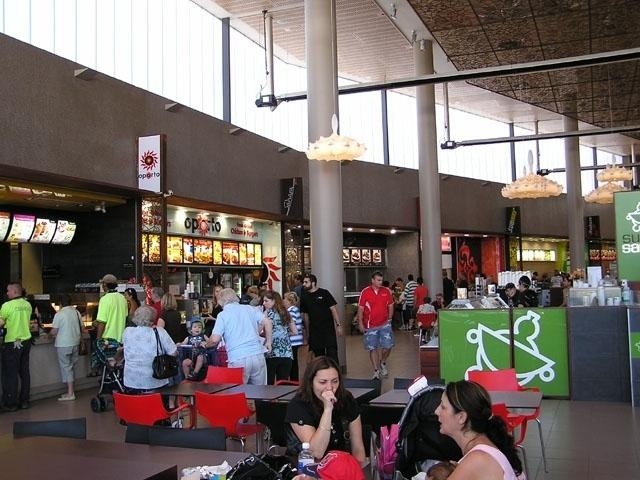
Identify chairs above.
[191,390,257,452]
[467,367,547,477]
[416,312,437,347]
[10,416,89,440]
[390,375,445,390]
[109,390,195,431]
[122,421,230,452]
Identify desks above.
[368,386,547,448]
[1,435,370,479]
[101,359,383,426]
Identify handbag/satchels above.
[151,325,178,380]
[227,446,296,480]
[76,311,88,355]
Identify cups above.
[598,286,620,307]
[184,281,194,298]
[498,270,531,288]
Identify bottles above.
[296,442,315,476]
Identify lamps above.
[304,0,367,166]
[595,3,634,180]
[582,183,634,204]
[499,38,565,200]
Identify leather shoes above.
[61,393,66,397]
[57,394,75,401]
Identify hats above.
[189,316,205,329]
[99,274,117,284]
[316,449,365,480]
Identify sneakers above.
[0,403,18,412]
[18,399,30,409]
[408,324,417,332]
[380,360,388,378]
[372,369,380,380]
[188,371,199,381]
[398,325,407,331]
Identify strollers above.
[85,335,125,413]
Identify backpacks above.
[375,422,401,476]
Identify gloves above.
[13,339,23,349]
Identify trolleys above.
[172,339,220,430]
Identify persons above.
[425,462,457,480]
[97,275,208,408]
[0,282,32,411]
[357,273,394,378]
[205,273,342,385]
[436,379,527,480]
[397,272,467,343]
[286,357,367,468]
[48,294,82,401]
[293,451,364,480]
[505,268,572,307]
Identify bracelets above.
[319,425,332,433]
